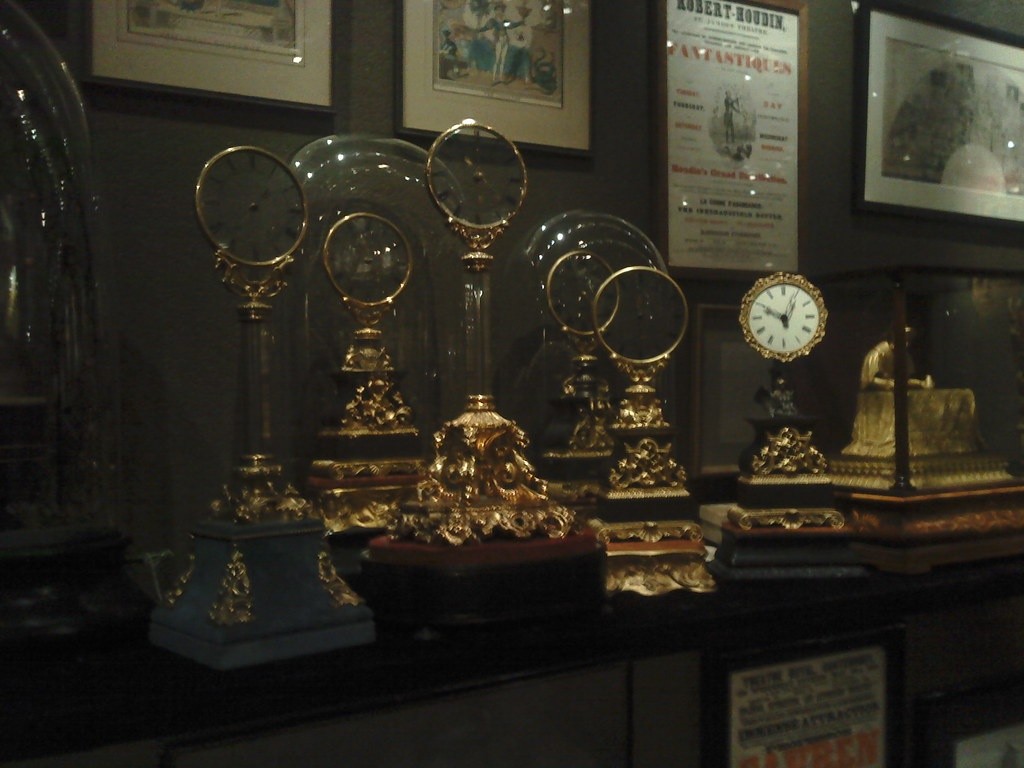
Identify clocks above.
[736,270,828,363]
